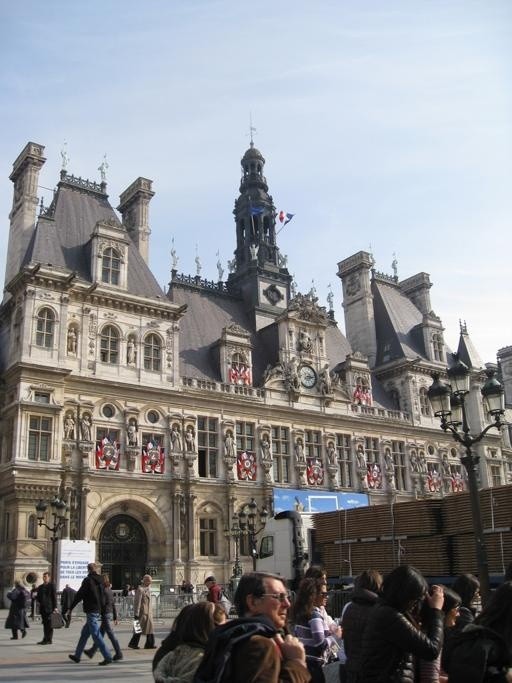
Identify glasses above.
[268,592,293,602]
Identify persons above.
[394,584,461,683]
[4,580,30,640]
[341,571,381,663]
[223,572,311,683]
[36,572,57,645]
[128,574,157,649]
[124,584,136,617]
[306,566,336,632]
[355,566,444,683]
[291,579,342,683]
[67,562,114,665]
[152,601,225,683]
[28,584,38,618]
[206,576,221,603]
[180,581,194,604]
[82,573,123,661]
[447,581,512,683]
[442,574,481,669]
[60,584,77,628]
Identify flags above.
[249,206,265,216]
[278,210,294,224]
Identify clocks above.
[297,363,317,388]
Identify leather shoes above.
[69,650,122,665]
[128,643,140,648]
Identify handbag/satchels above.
[46,613,63,628]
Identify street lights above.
[35,492,73,583]
[424,357,512,612]
[244,498,268,571]
[223,512,242,591]
